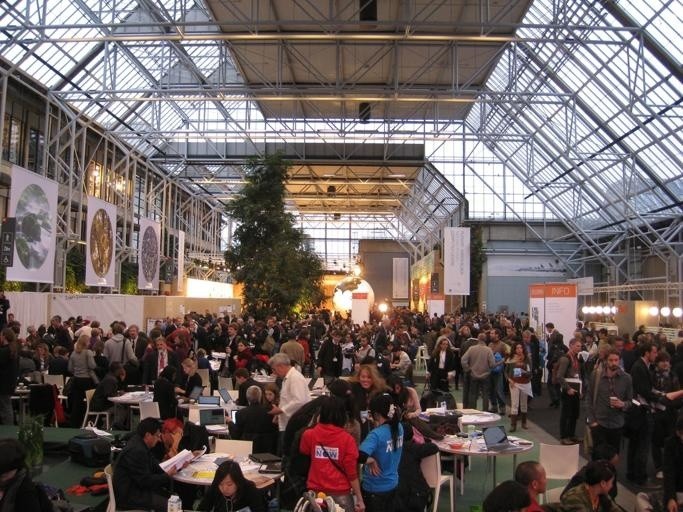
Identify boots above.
[521,413,528,427]
[510,415,517,432]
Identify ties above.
[160,352,164,368]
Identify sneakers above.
[634,482,661,489]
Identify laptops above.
[231,410,238,424]
[197,396,220,407]
[482,426,522,451]
[218,388,238,404]
[249,453,281,462]
[181,386,204,404]
[199,408,228,431]
[259,456,284,473]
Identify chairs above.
[0,344,660,510]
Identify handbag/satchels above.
[178,422,210,454]
[51,405,65,424]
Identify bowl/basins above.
[448,443,463,448]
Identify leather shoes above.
[560,438,576,444]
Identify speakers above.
[334,213,340,220]
[328,186,335,192]
[359,103,371,119]
[359,0,378,21]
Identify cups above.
[609,397,617,407]
[440,402,446,411]
[467,424,475,440]
